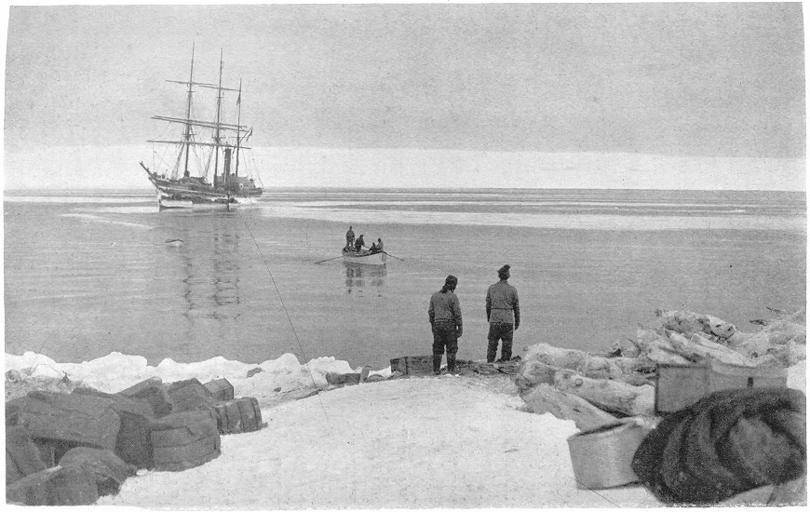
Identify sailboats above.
[135,39,254,207]
[212,76,264,198]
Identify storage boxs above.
[657,362,788,414]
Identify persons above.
[427,274,463,375]
[369,242,378,254]
[376,238,384,250]
[484,263,522,364]
[354,234,366,252]
[344,223,357,248]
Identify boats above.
[341,246,388,268]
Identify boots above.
[433,354,441,375]
[446,352,455,372]
